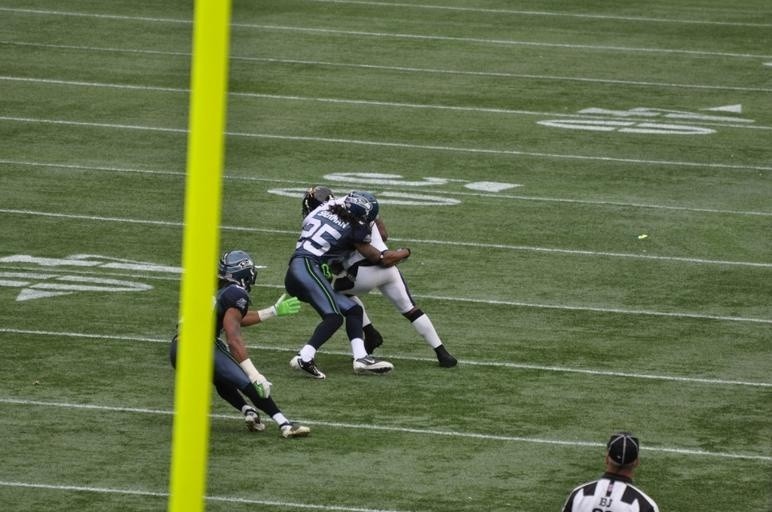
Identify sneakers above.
[280,421,311,438]
[364,333,384,354]
[435,352,458,368]
[353,357,393,374]
[289,354,326,380]
[244,414,265,431]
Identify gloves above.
[250,370,272,399]
[271,292,301,317]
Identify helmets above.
[218,251,257,292]
[302,185,334,217]
[342,192,379,226]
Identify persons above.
[283,190,411,379]
[563,433,659,512]
[169,251,310,437]
[298,186,459,367]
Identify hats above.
[606,431,640,466]
